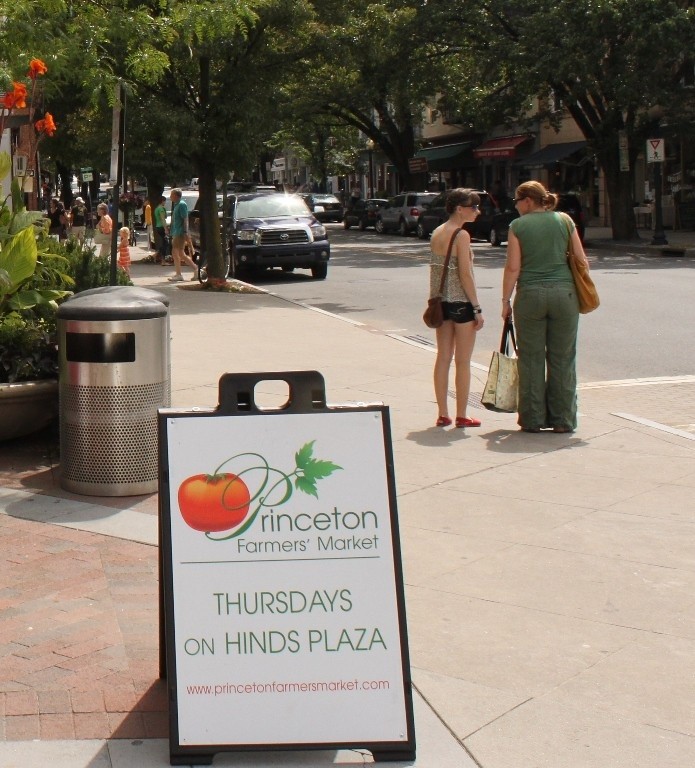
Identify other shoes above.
[520,425,540,432]
[191,267,200,281]
[436,416,452,426]
[168,275,184,281]
[455,416,481,427]
[554,423,573,433]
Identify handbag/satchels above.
[480,316,517,413]
[93,233,110,244]
[568,253,601,314]
[422,296,443,328]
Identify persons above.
[118,227,131,281]
[339,182,361,208]
[148,196,170,265]
[96,203,112,260]
[46,197,87,250]
[168,188,198,281]
[502,180,585,433]
[430,187,485,428]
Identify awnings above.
[473,133,530,158]
[514,141,594,166]
[415,140,475,170]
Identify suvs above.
[374,191,440,236]
[217,194,331,280]
[487,196,585,246]
[415,189,500,242]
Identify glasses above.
[512,197,525,204]
[460,203,479,211]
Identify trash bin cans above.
[60,286,171,496]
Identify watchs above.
[473,309,481,314]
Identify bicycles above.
[196,232,231,284]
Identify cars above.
[342,198,386,232]
[67,177,277,260]
[303,193,345,224]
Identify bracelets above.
[183,230,189,233]
[472,304,480,308]
[502,298,510,303]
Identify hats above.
[75,197,85,204]
